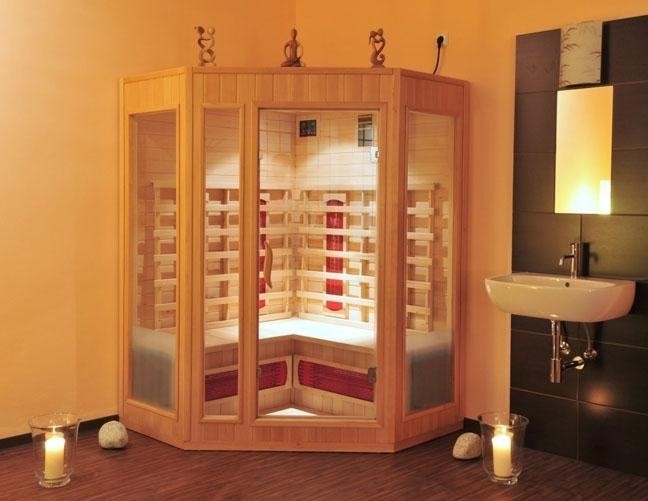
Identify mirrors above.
[553,84,615,216]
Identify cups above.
[478,410,530,486]
[27,412,81,489]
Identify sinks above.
[484,271,637,326]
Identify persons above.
[280,28,305,68]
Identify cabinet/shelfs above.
[119,64,465,454]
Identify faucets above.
[557,241,581,279]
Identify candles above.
[494,423,510,477]
[44,426,64,478]
